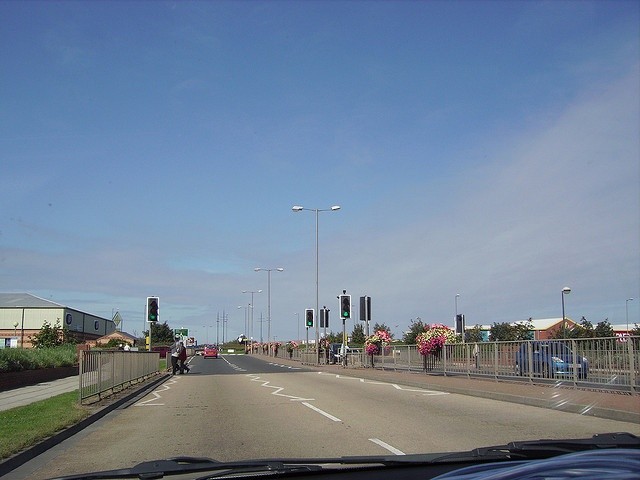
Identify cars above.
[204,344,218,359]
[515,341,588,380]
[195,348,204,356]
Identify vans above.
[330,343,342,362]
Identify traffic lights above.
[146,297,160,323]
[339,295,351,319]
[305,309,314,328]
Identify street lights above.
[292,205,341,347]
[455,293,460,364]
[626,298,633,334]
[561,286,571,339]
[237,305,254,353]
[203,325,213,344]
[254,267,284,342]
[242,290,263,354]
[216,320,228,344]
[294,312,299,340]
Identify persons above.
[178,342,191,375]
[472,341,482,373]
[170,338,180,375]
